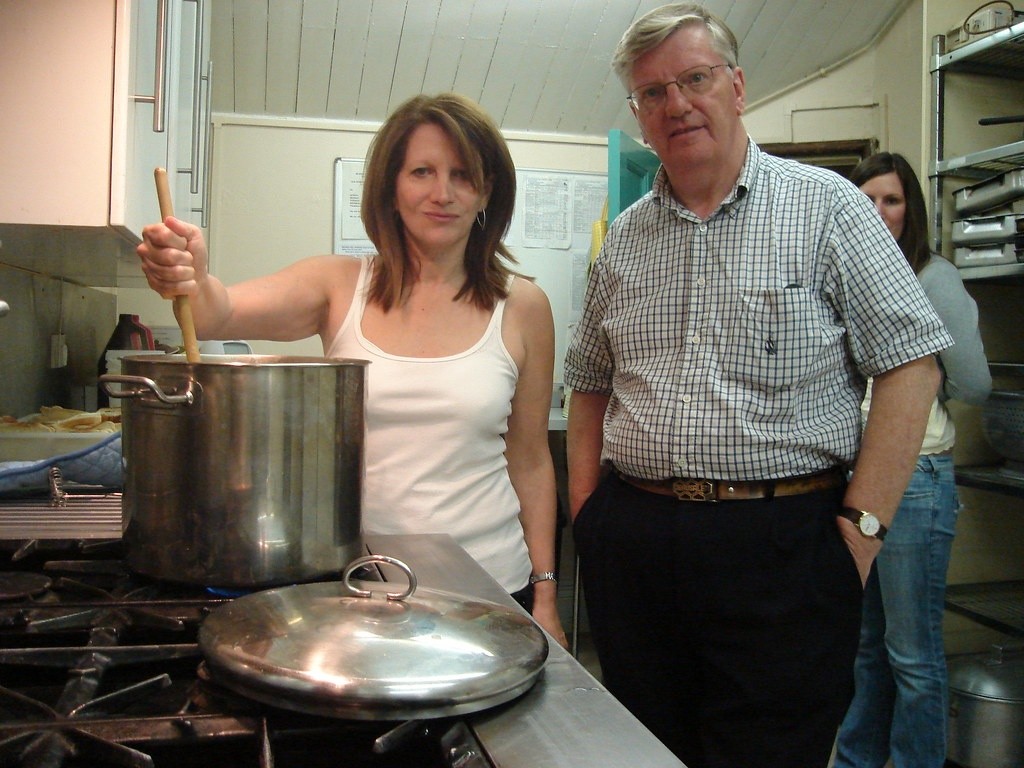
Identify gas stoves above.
[0,539,495,768]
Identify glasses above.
[626,64,733,111]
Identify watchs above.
[838,507,888,541]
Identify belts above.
[611,464,839,503]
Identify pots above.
[101,352,373,588]
[942,644,1024,768]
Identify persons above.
[562,2,954,768]
[832,152,992,768]
[138,93,567,651]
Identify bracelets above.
[529,572,557,584]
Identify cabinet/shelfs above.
[0,0,215,288]
[927,19,1024,643]
